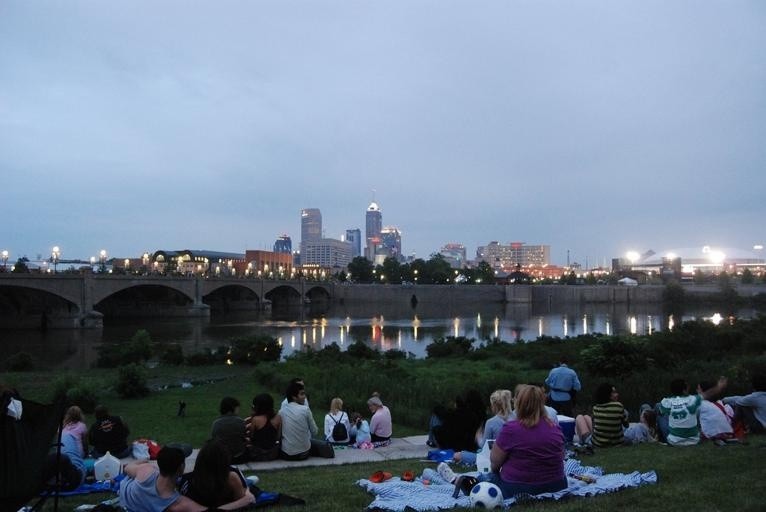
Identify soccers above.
[469,481,505,512]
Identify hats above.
[157,442,192,471]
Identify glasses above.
[511,398,517,402]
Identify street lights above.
[52,246,60,273]
[2,250,9,271]
[143,254,150,275]
[99,250,108,272]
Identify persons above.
[640,373,729,447]
[281,375,310,411]
[421,385,568,499]
[544,354,581,419]
[349,412,372,446]
[624,408,658,444]
[207,397,255,464]
[324,397,352,447]
[693,378,735,443]
[368,396,393,443]
[52,407,90,458]
[511,382,562,426]
[248,392,282,462]
[87,403,133,461]
[180,438,259,508]
[425,386,490,454]
[278,383,318,461]
[115,443,258,511]
[480,389,516,453]
[576,382,629,448]
[721,375,766,436]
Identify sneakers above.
[438,462,457,482]
[422,467,444,485]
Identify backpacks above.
[325,411,348,442]
